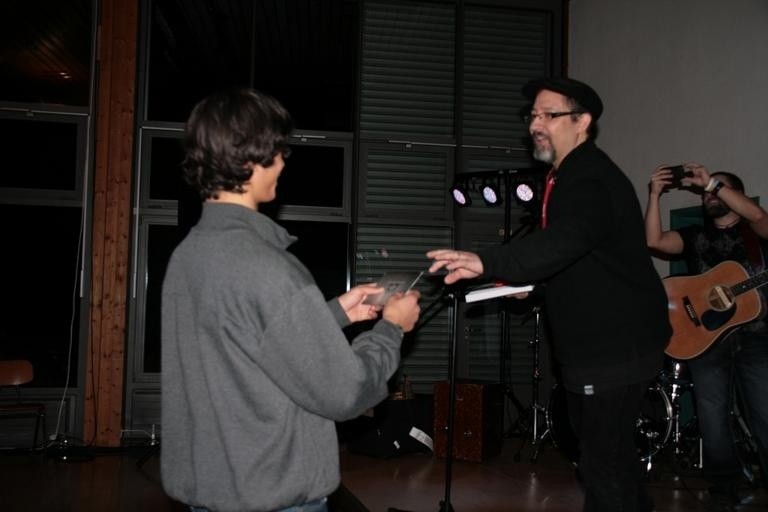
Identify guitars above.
[660,260,767,361]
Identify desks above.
[1,433,190,512]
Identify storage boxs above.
[432,378,506,463]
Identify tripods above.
[504,311,548,465]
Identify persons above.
[159,86,422,512]
[426,75,673,512]
[644,161,768,511]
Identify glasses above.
[524,110,588,122]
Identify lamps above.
[479,183,502,208]
[512,179,537,207]
[450,182,473,208]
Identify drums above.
[544,377,680,466]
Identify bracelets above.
[704,177,725,195]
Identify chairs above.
[1,360,46,455]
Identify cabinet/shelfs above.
[345,395,418,461]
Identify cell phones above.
[660,165,686,182]
